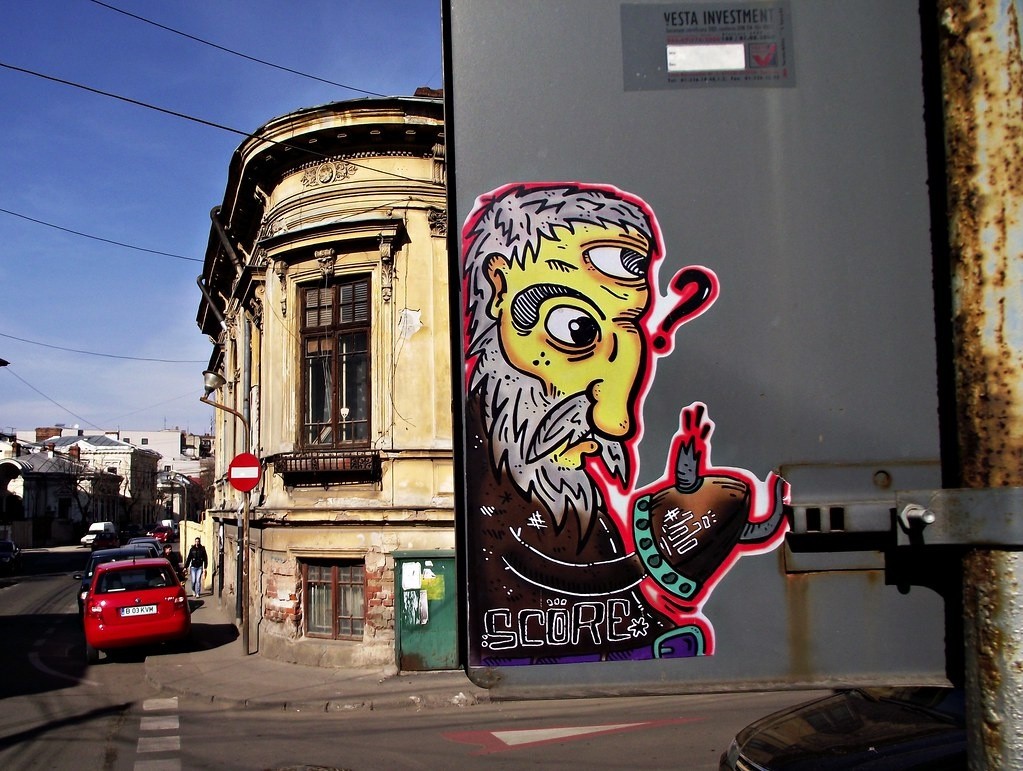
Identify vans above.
[80,522,115,547]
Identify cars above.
[0,539,22,574]
[80,545,191,664]
[74,546,160,618]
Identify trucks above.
[161,519,178,536]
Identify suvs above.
[91,533,119,552]
[146,526,175,543]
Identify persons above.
[182,538,208,597]
[160,544,184,582]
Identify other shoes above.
[192,590,196,597]
[196,593,199,598]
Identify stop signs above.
[227,452,261,493]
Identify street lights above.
[163,474,187,563]
